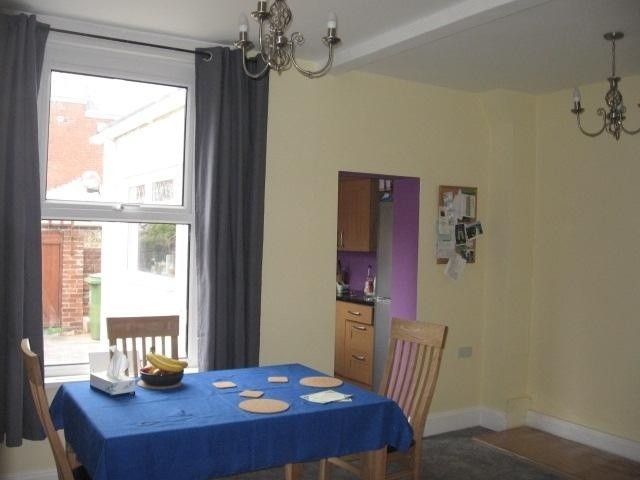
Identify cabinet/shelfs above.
[334,299,374,391]
[335,176,376,252]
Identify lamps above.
[570,31,640,141]
[233,0,341,79]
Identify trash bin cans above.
[84,273,101,341]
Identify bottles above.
[364,265,374,297]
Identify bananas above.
[147,351,188,374]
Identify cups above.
[350,287,358,296]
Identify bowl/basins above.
[139,367,184,386]
[337,284,352,294]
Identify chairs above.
[318,317,448,480]
[20,338,90,480]
[106,315,180,377]
